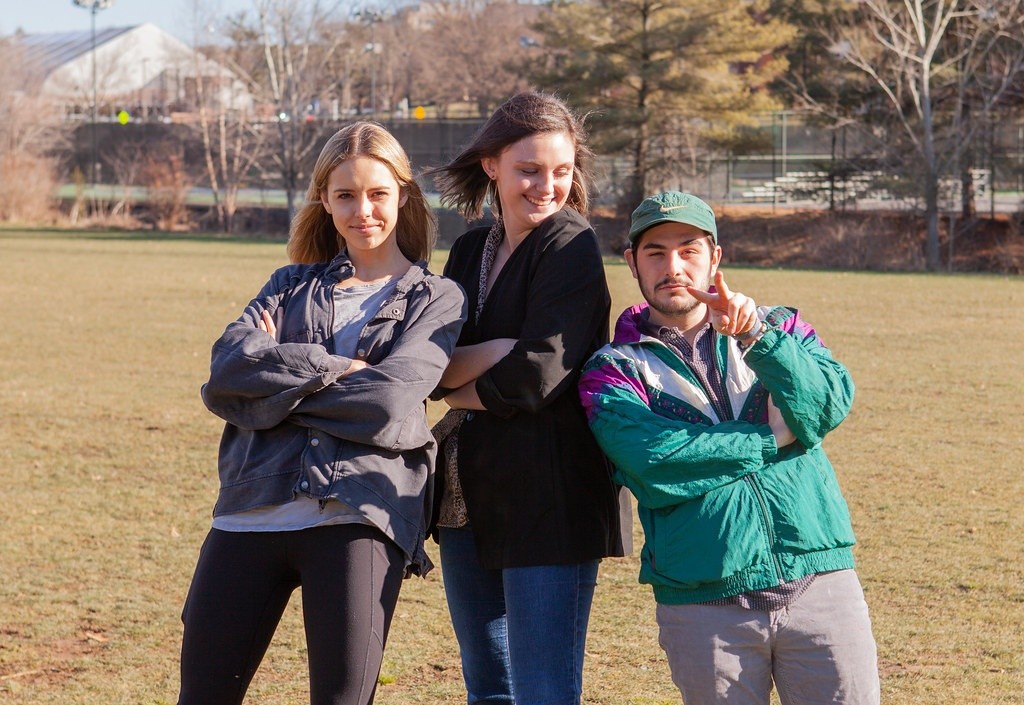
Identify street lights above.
[73,0,111,185]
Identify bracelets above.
[732,317,760,340]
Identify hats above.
[628,190,722,247]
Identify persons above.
[175,121,468,705]
[425,93,631,705]
[578,193,882,705]
